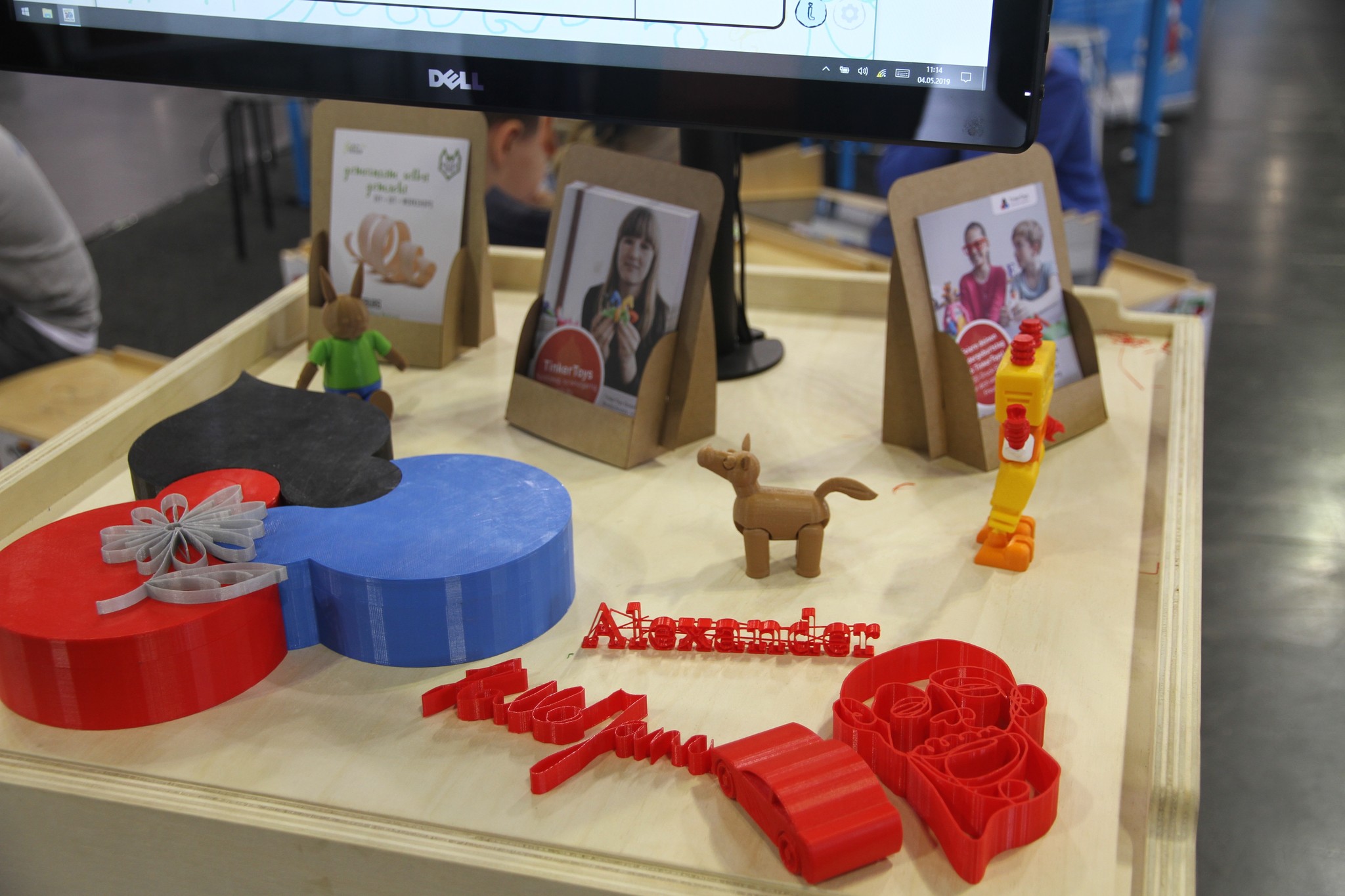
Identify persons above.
[482,110,552,248]
[1,126,101,382]
[582,207,671,396]
[998,219,1062,330]
[959,222,1012,327]
[870,34,1122,286]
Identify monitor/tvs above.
[0,0,1051,153]
